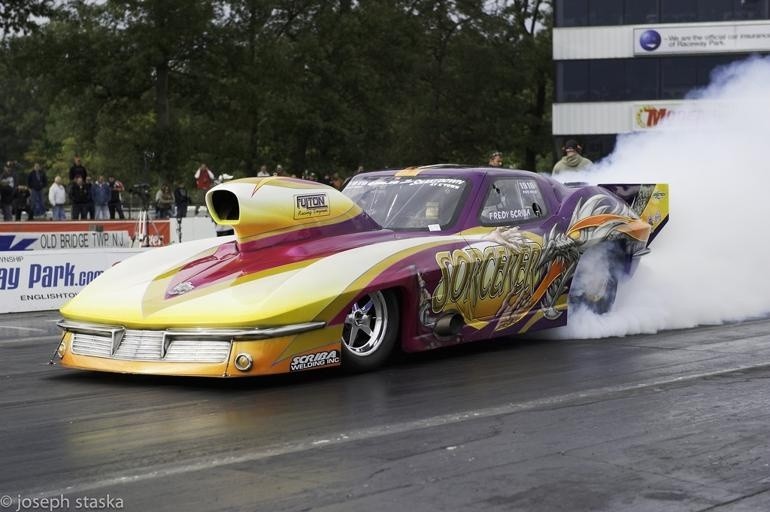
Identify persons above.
[488,152,503,168]
[552,140,594,178]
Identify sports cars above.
[53,158,672,389]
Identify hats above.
[565,139,579,152]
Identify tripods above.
[131,211,161,247]
